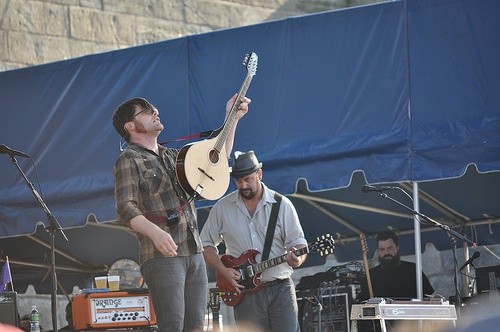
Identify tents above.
[0,0,500,293]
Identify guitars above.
[359,233,387,332]
[215,234,336,307]
[175,51,259,202]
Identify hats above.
[230,149,263,178]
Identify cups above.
[108,276,120,291]
[94,276,107,289]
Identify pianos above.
[350,304,457,321]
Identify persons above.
[112,93,251,332]
[357,230,435,332]
[200,150,309,332]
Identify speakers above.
[0,292,18,327]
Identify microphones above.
[361,185,400,193]
[0,144,29,158]
[459,251,480,271]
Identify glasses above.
[127,106,159,122]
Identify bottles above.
[29,306,41,332]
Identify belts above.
[259,278,290,288]
